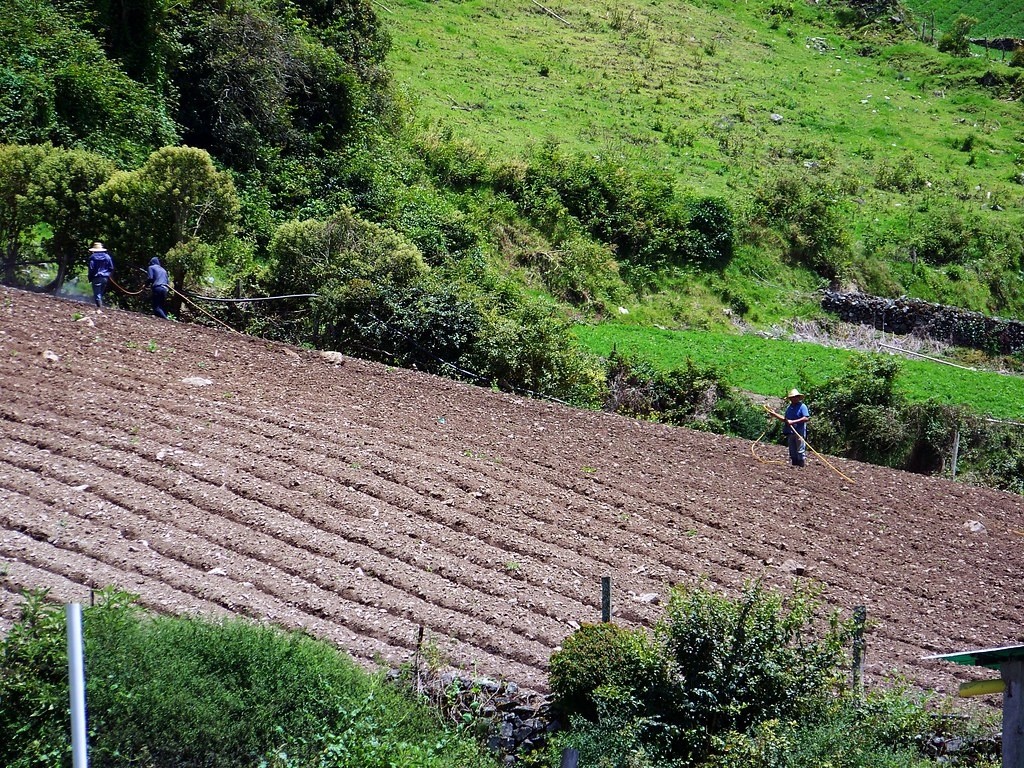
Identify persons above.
[89,242,114,308]
[769,389,810,467]
[144,256,169,320]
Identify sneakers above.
[95,298,102,306]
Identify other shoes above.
[791,459,805,466]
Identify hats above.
[89,242,107,252]
[786,389,804,400]
